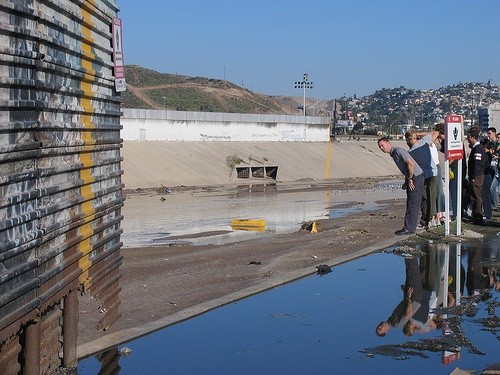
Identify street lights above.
[294,72,314,116]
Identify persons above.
[377,123,500,235]
[376,244,500,336]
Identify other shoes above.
[394,227,415,235]
[429,221,437,228]
[435,221,442,226]
[471,220,483,225]
[483,217,487,220]
[416,223,428,229]
